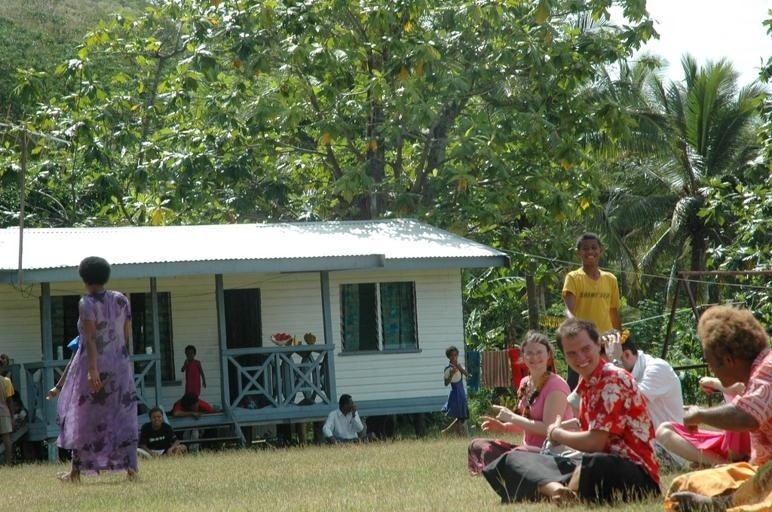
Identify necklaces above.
[515,372,550,418]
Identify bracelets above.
[548,426,558,441]
[192,411,195,417]
[612,359,624,366]
[56,383,63,391]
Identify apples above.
[274,333,288,341]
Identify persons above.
[562,232,623,392]
[323,394,364,446]
[181,345,206,398]
[137,407,188,460]
[654,375,751,469]
[466,332,574,477]
[440,346,470,439]
[1,354,12,382]
[482,317,661,507]
[1,357,16,467]
[566,328,687,478]
[54,256,139,483]
[164,393,213,418]
[6,390,28,433]
[46,333,100,477]
[662,304,772,511]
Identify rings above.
[96,381,98,384]
